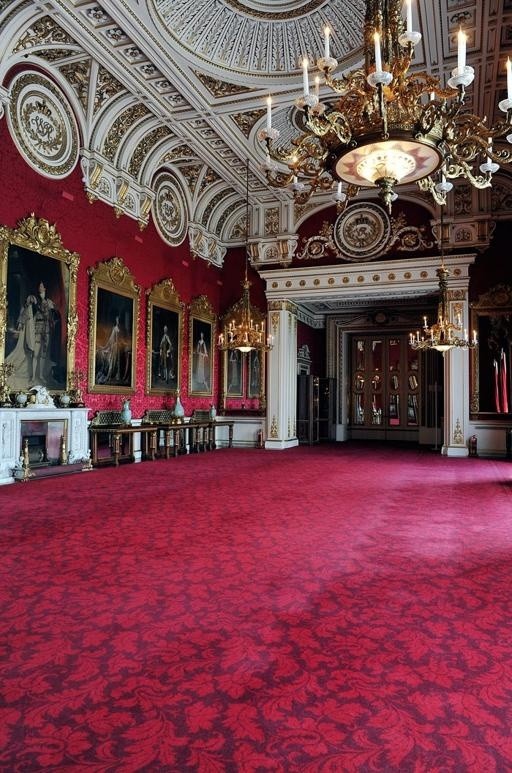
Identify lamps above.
[410,206,478,354]
[216,158,274,354]
[257,1,512,213]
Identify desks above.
[149,421,209,460]
[188,420,235,452]
[88,424,158,467]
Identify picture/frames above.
[146,277,185,394]
[188,294,215,397]
[87,256,140,395]
[469,283,512,416]
[248,306,263,398]
[1,212,80,396]
[221,299,244,398]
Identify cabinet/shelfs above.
[350,335,385,442]
[298,375,336,446]
[386,329,425,442]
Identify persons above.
[5,279,63,390]
[194,332,207,390]
[229,348,241,391]
[98,314,131,385]
[159,323,174,385]
[250,348,260,388]
